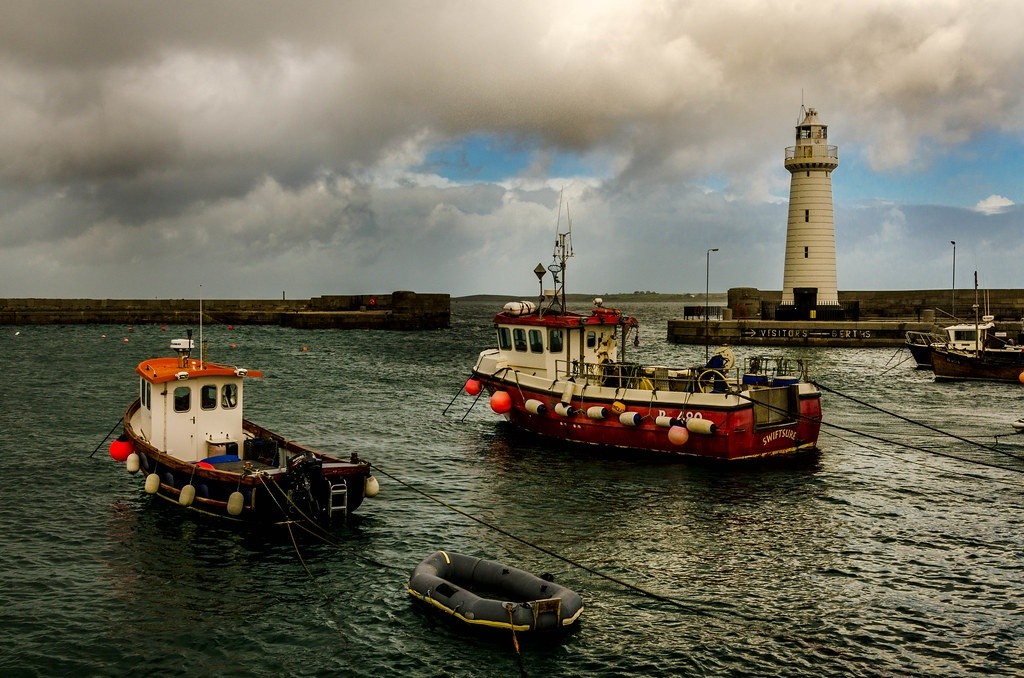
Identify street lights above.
[706,248,719,319]
[951,241,956,320]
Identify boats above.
[407,548,585,643]
[120,286,370,538]
[905,271,1024,382]
[469,188,822,462]
